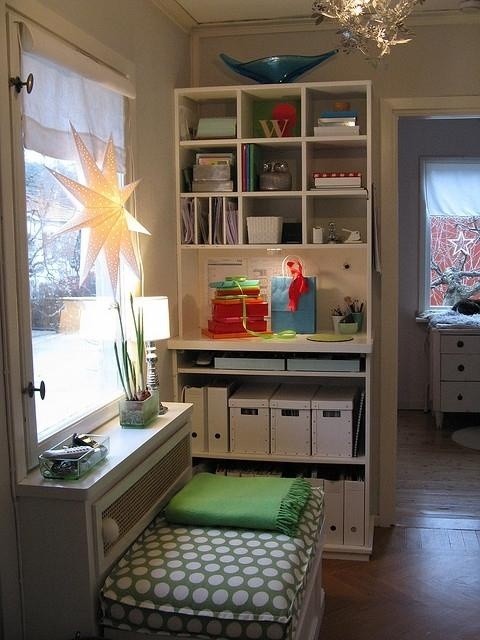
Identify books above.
[242,143,263,192]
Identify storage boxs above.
[311,384,359,458]
[270,383,320,456]
[229,381,281,455]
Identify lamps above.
[122,296,170,416]
[312,0,424,71]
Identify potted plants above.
[113,293,160,428]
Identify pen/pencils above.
[328,296,366,316]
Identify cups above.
[332,315,345,333]
[352,312,364,330]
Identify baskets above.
[247,217,283,243]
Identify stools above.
[100,473,326,640]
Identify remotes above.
[43,445,93,458]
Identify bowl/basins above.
[338,323,358,334]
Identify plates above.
[219,48,340,84]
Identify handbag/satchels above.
[270,255,316,334]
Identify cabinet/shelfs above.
[429,327,480,429]
[165,79,380,565]
